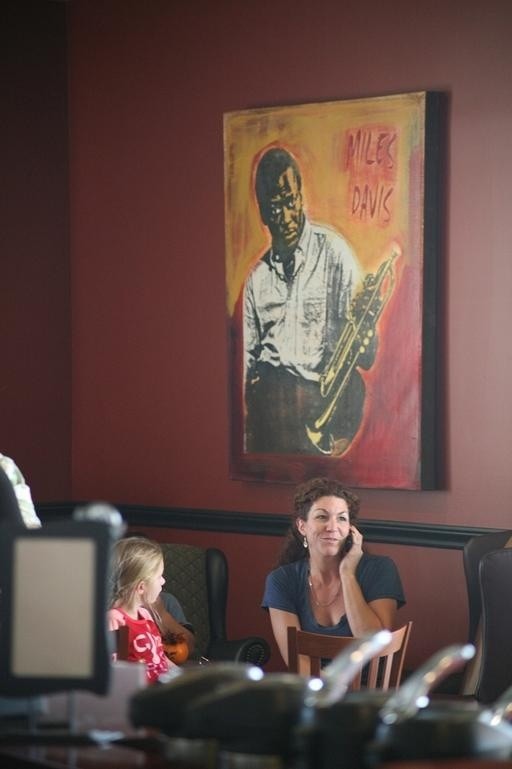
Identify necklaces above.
[306,574,343,609]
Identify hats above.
[72,500,128,542]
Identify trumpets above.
[304,248,401,458]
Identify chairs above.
[399,528,511,710]
[157,542,271,668]
[286,621,414,690]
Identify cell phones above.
[339,535,353,554]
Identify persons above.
[98,535,181,690]
[72,501,197,662]
[258,474,407,686]
[233,143,381,456]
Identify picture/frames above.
[0,521,111,700]
